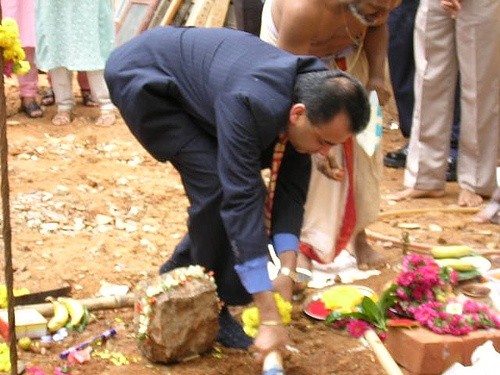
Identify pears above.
[18,336,31,350]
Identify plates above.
[303,286,377,321]
[435,257,492,280]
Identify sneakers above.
[215,308,255,350]
[159,260,178,276]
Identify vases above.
[3,73,22,118]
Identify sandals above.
[41,87,55,106]
[21,96,43,117]
[81,88,99,107]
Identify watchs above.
[276,266,300,290]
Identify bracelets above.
[258,319,284,327]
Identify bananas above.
[431,245,474,258]
[438,261,476,270]
[45,296,70,331]
[57,296,84,327]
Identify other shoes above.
[384,143,409,166]
[96,111,116,127]
[53,111,71,126]
[444,159,456,180]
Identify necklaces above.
[339,0,366,43]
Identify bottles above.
[264,351,283,375]
[457,285,492,308]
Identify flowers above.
[325,254,500,343]
[0,17,31,76]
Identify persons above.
[382,0,500,207]
[256,0,402,294]
[0,0,118,128]
[103,25,371,360]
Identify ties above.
[263,130,290,237]
